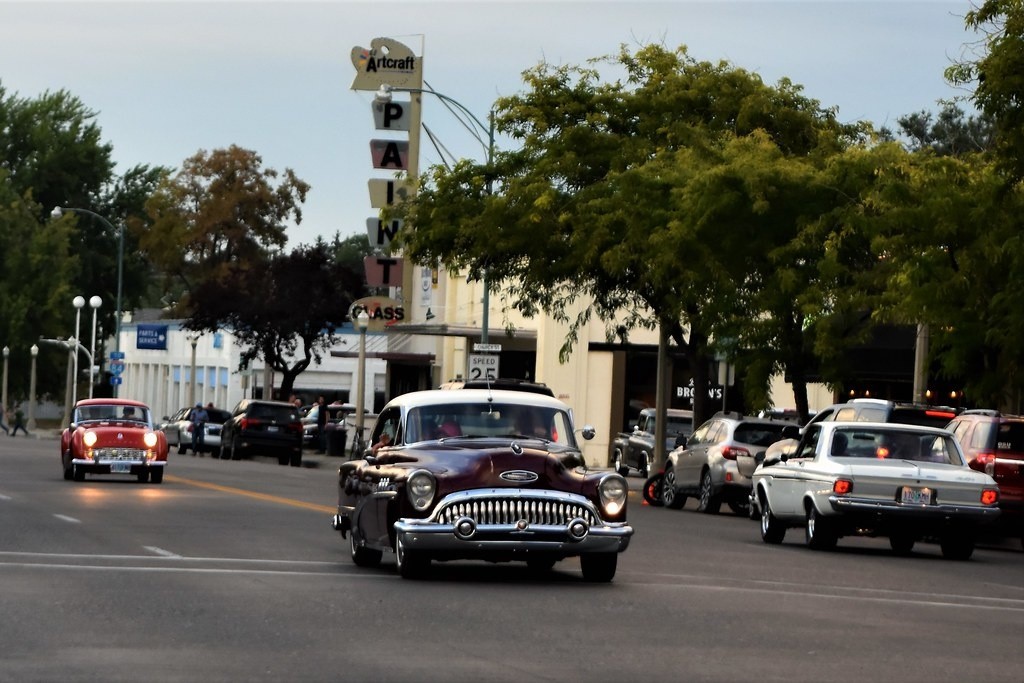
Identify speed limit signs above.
[468,354,500,384]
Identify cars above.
[330,378,634,582]
[159,406,231,458]
[299,403,369,450]
[747,421,1000,561]
[62,398,169,484]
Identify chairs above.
[437,420,464,438]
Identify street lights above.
[39,296,102,408]
[375,83,494,356]
[2,346,9,433]
[50,206,123,398]
[27,344,38,430]
[357,310,369,447]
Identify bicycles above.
[347,422,370,461]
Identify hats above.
[196,401,203,407]
[438,419,463,437]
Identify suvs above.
[220,400,304,467]
[613,398,1024,540]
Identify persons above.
[122,407,135,419]
[834,433,849,456]
[315,396,330,454]
[509,411,547,438]
[336,411,343,420]
[290,394,343,419]
[373,422,403,454]
[0,401,9,436]
[89,407,100,419]
[191,402,209,457]
[11,406,28,436]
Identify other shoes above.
[314,449,326,455]
[199,452,204,457]
[191,452,196,456]
